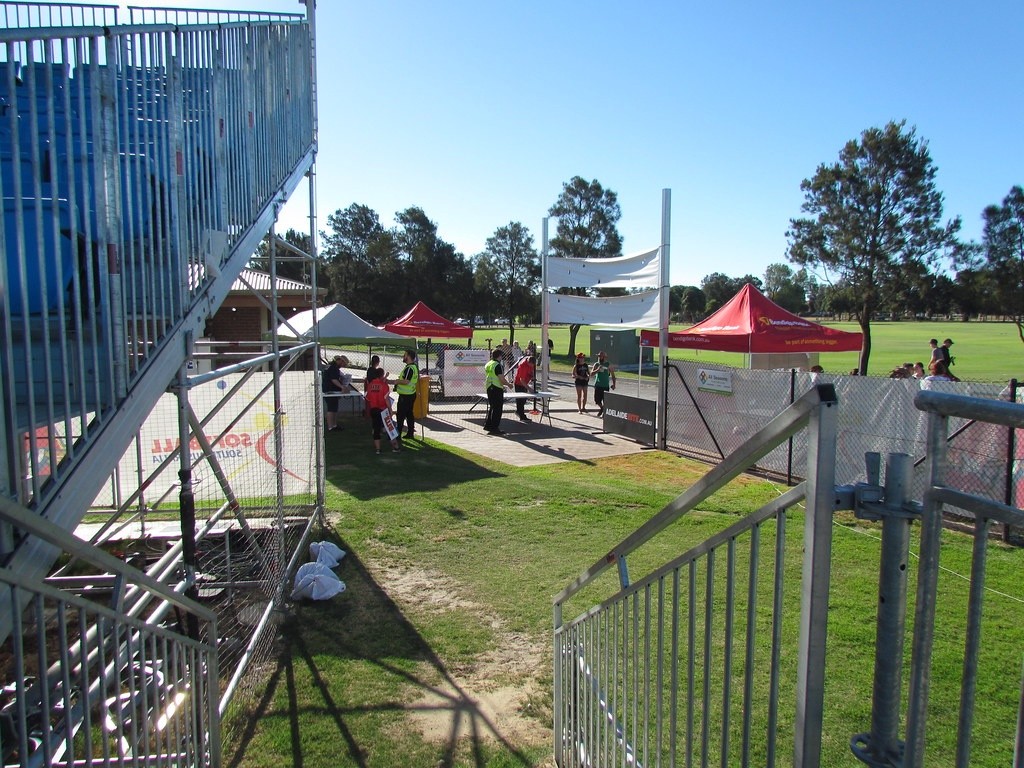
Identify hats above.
[334,356,349,365]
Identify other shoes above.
[579,410,582,414]
[392,447,401,453]
[490,428,504,435]
[515,410,526,420]
[402,433,414,439]
[375,448,381,454]
[328,427,339,432]
[484,425,492,430]
[520,415,532,422]
[334,425,344,431]
[582,408,588,413]
[597,407,603,417]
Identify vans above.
[872,311,886,321]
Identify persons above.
[810,338,960,382]
[366,355,390,384]
[366,368,404,455]
[393,350,419,439]
[572,352,590,414]
[322,354,349,432]
[514,355,536,421]
[590,350,616,419]
[495,333,554,383]
[483,349,513,435]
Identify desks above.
[476,392,560,428]
[323,390,362,416]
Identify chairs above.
[419,368,443,393]
[0,62,247,329]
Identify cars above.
[810,311,834,317]
[453,316,515,325]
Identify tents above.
[638,282,864,411]
[276,303,424,442]
[378,301,473,383]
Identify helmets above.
[596,351,608,359]
[577,353,585,358]
